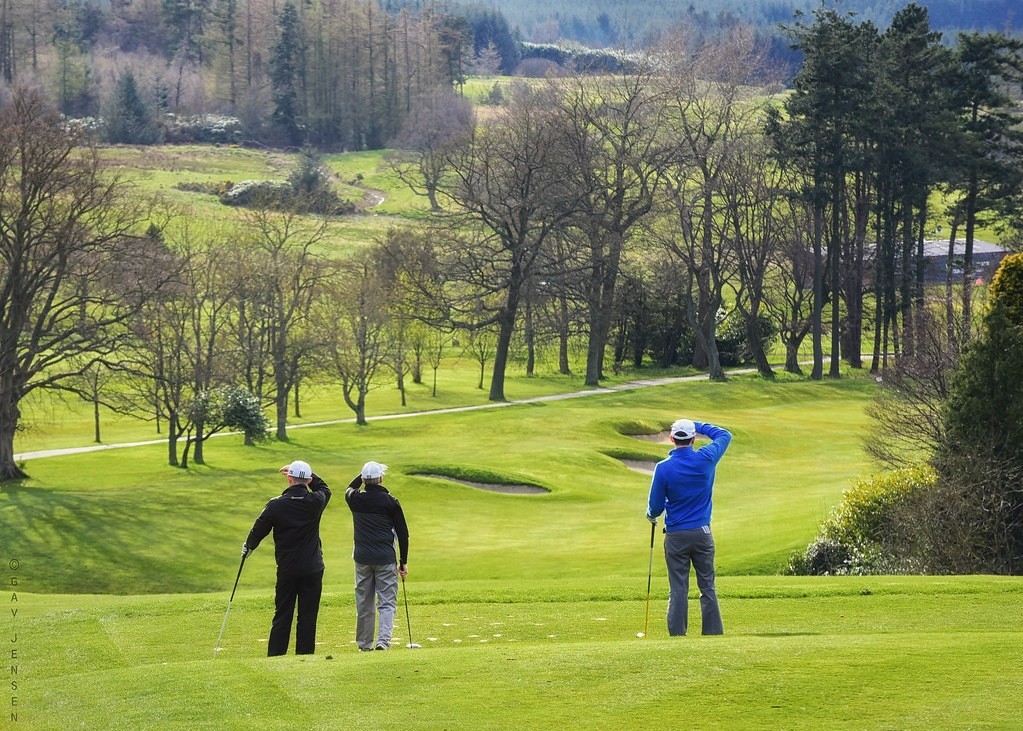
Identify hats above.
[670,419,696,440]
[361,461,389,479]
[286,461,312,479]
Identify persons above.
[645,419,732,637]
[241,460,332,657]
[344,462,409,651]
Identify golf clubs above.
[213,545,248,657]
[399,558,419,649]
[637,517,656,638]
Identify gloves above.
[240,542,253,558]
[645,512,659,526]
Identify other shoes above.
[358,646,373,651]
[375,642,390,650]
